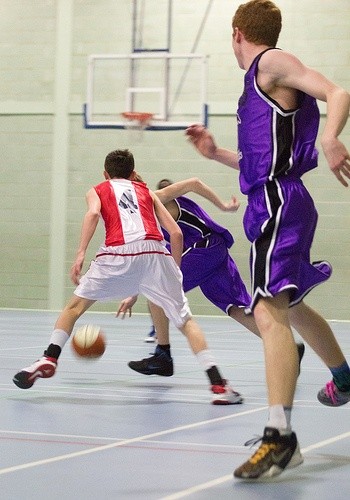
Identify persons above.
[12,148,245,406]
[115,174,305,378]
[183,0,350,482]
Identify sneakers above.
[209,385,244,404]
[297,343,305,380]
[232,426,304,482]
[13,355,57,390]
[317,378,350,406]
[145,326,158,341]
[128,352,174,376]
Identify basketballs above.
[73,325,107,358]
